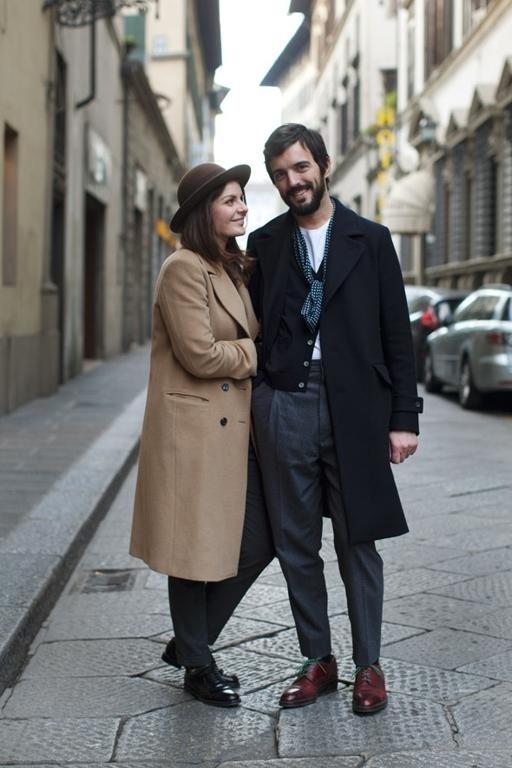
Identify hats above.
[169,161,251,234]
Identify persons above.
[127,162,261,706]
[244,122,423,718]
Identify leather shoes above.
[161,636,238,687]
[353,662,387,712]
[183,664,240,708]
[279,655,338,709]
